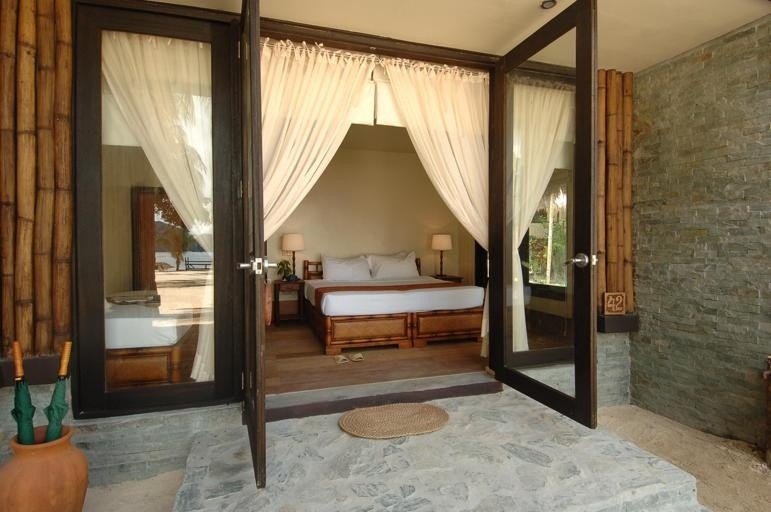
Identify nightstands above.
[274,280,305,326]
[430,274,464,283]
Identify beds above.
[303,258,484,356]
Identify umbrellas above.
[11,341,37,445]
[43,340,73,443]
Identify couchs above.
[104,289,200,390]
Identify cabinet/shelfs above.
[131,185,189,290]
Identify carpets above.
[337,402,450,440]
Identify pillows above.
[323,254,371,281]
[368,251,407,269]
[372,250,420,281]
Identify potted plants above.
[277,260,291,281]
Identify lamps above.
[281,232,305,279]
[432,234,453,277]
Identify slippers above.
[334,354,350,365]
[349,352,365,362]
